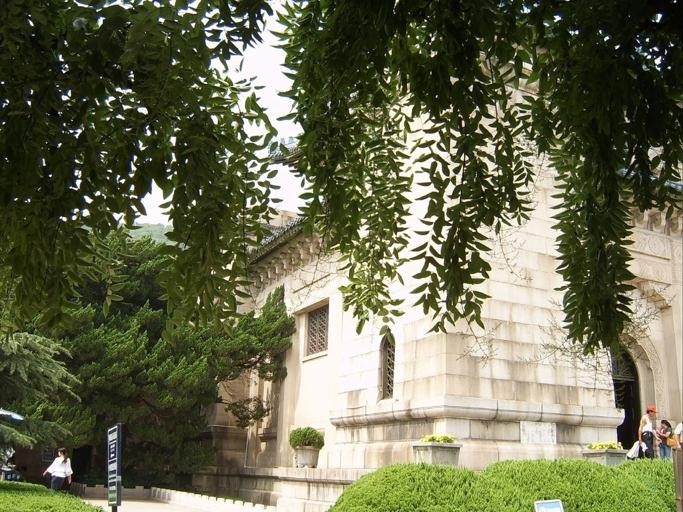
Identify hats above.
[647,405,659,414]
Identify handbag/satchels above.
[666,428,678,447]
[642,431,653,442]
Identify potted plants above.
[286,424,325,469]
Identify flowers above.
[585,439,624,449]
[417,431,459,444]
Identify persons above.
[638,404,661,459]
[42,448,74,491]
[656,420,673,460]
[673,421,683,449]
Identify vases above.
[412,444,463,468]
[580,448,629,467]
